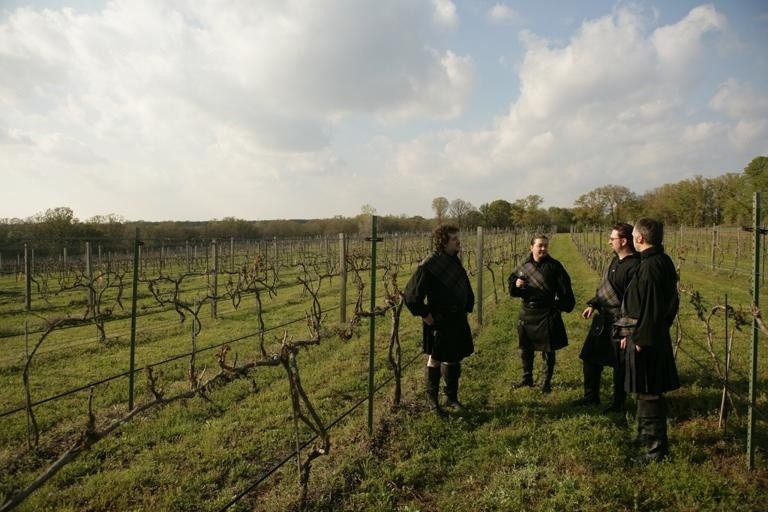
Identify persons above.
[402,225,475,416]
[572,223,640,408]
[508,235,575,394]
[613,217,679,466]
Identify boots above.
[425,363,449,417]
[604,371,625,412]
[629,399,671,463]
[513,359,533,388]
[439,362,470,410]
[572,368,601,404]
[540,360,552,392]
